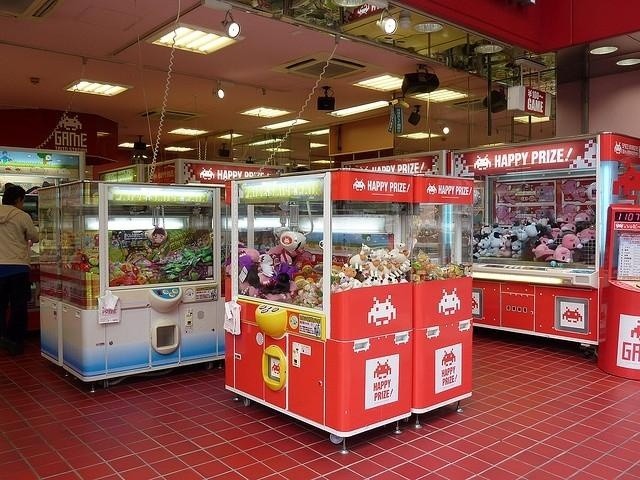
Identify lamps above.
[220,9,241,40]
[212,80,224,100]
[375,8,399,35]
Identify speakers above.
[402,73,439,97]
[408,112,420,125]
[318,97,335,109]
[134,143,146,150]
[483,90,507,113]
[220,150,228,156]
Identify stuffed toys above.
[473,181,596,263]
[224,240,472,310]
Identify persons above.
[0,183,39,356]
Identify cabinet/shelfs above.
[1,132,640,455]
